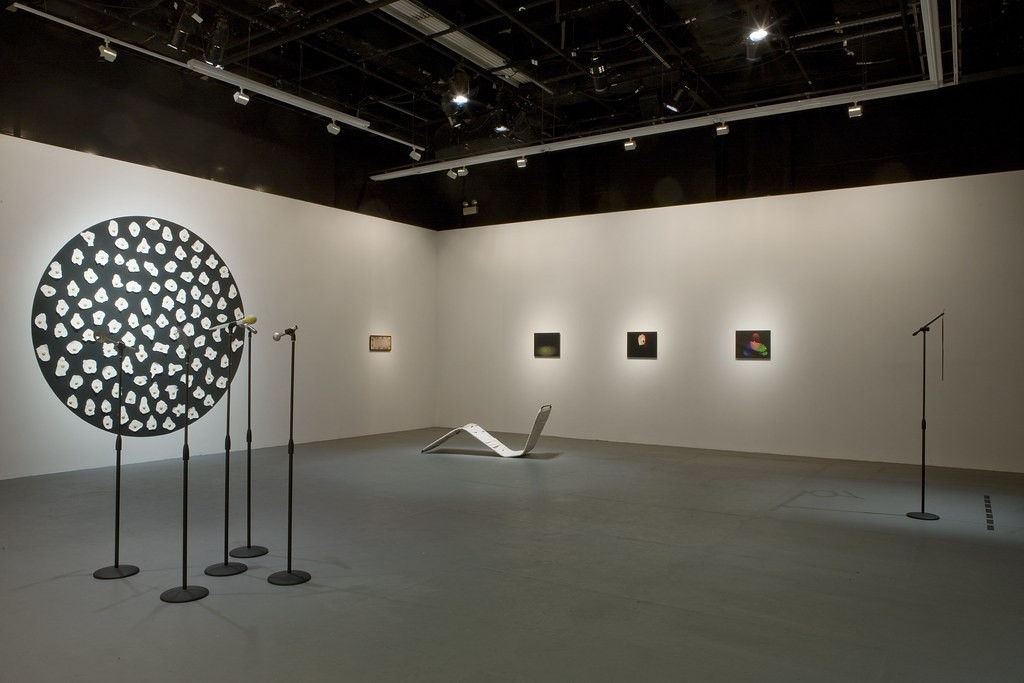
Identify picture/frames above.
[369,334,391,352]
[627,332,657,358]
[534,333,560,358]
[736,331,771,360]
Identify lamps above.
[586,53,609,93]
[624,138,637,151]
[446,169,457,179]
[462,200,479,215]
[327,118,340,135]
[716,121,729,136]
[440,69,470,129]
[848,101,863,118]
[517,156,527,168]
[458,166,468,176]
[665,82,691,113]
[491,87,511,133]
[98,37,117,62]
[233,86,250,105]
[410,147,422,161]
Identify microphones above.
[210,315,257,334]
[94,332,136,352]
[272,325,298,341]
